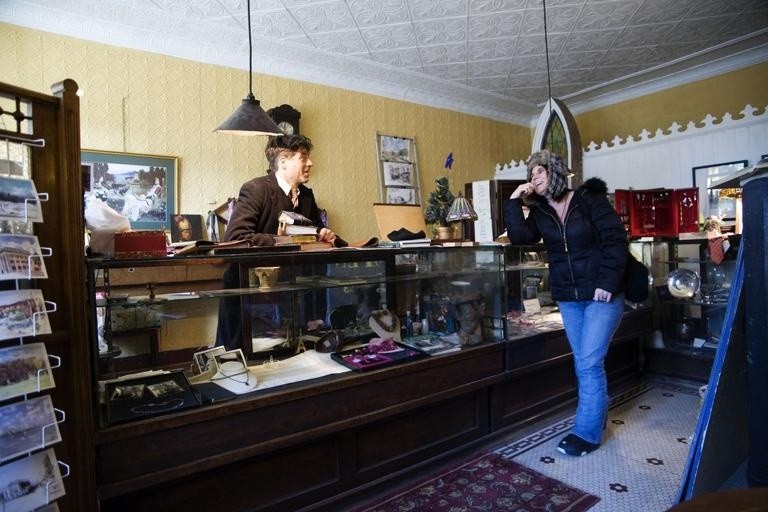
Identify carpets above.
[354,448,601,512]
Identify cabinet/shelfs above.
[0,74,88,512]
[501,232,648,437]
[372,131,425,208]
[645,222,740,393]
[82,240,502,512]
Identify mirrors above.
[691,159,746,234]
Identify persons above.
[503,148,629,460]
[212,133,349,349]
[173,215,194,241]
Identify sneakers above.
[555,431,599,458]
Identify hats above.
[526,150,568,198]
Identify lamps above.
[214,1,284,139]
[445,190,480,238]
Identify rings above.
[602,296,607,299]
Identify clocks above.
[267,103,303,140]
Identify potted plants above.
[424,176,457,239]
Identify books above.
[376,237,432,248]
[275,209,333,251]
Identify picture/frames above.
[80,147,182,237]
